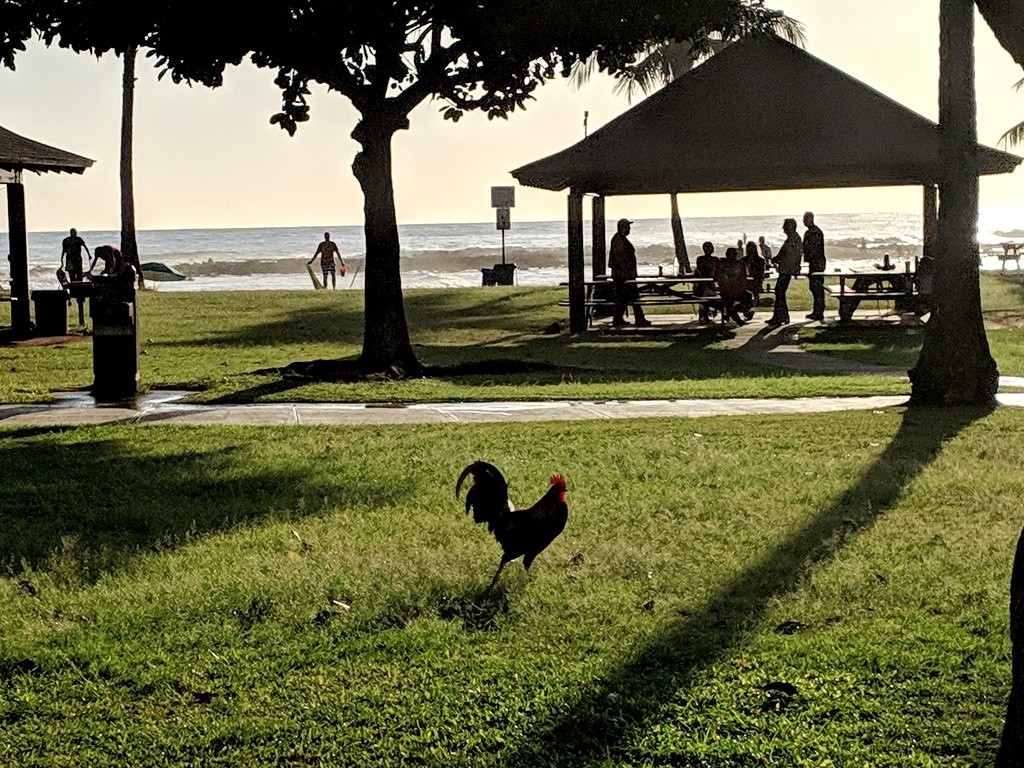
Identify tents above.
[0,126,97,329]
[507,25,1024,332]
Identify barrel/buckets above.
[481,268,497,287]
[340,267,346,276]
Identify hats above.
[617,219,633,232]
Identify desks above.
[561,276,755,330]
[811,271,915,324]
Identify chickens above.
[456,461,568,575]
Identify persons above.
[693,233,772,326]
[764,219,803,325]
[61,228,92,283]
[90,245,122,275]
[608,218,652,326]
[802,212,826,321]
[308,232,344,290]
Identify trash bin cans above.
[84,277,140,402]
[480,263,517,286]
[31,289,68,334]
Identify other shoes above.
[766,315,790,325]
[613,318,630,326]
[636,317,651,326]
[748,311,754,319]
[805,312,824,320]
[737,320,745,326]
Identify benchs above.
[826,284,919,326]
[557,290,755,331]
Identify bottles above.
[678,261,686,276]
[905,262,913,297]
